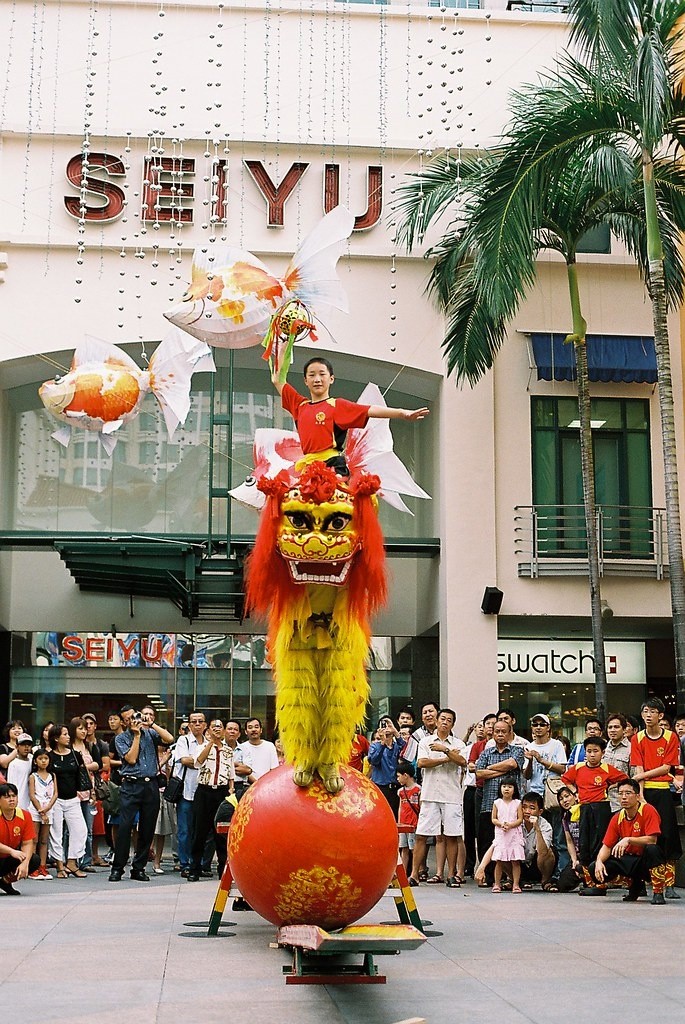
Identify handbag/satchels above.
[92,801,105,835]
[93,769,111,800]
[78,765,93,791]
[163,777,184,803]
[157,774,167,787]
[544,775,578,810]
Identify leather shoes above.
[109,867,149,881]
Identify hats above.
[82,713,96,722]
[15,733,33,745]
[531,713,550,725]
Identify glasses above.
[618,792,639,797]
[532,722,550,727]
[587,726,602,732]
[192,719,204,725]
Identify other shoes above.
[0,878,20,895]
[152,853,235,884]
[575,874,681,905]
[79,851,136,873]
[27,865,53,880]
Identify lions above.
[245,469,379,790]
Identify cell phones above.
[216,720,220,728]
[381,720,386,729]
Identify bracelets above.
[151,722,154,726]
[547,763,552,769]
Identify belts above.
[199,783,229,790]
[376,783,400,790]
[126,777,156,783]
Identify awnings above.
[528,333,659,394]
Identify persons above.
[0,704,288,913]
[344,694,685,904]
[271,338,430,475]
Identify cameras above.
[132,713,143,722]
[182,726,189,734]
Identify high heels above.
[57,865,88,878]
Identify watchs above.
[444,748,449,754]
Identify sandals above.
[405,865,560,904]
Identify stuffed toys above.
[242,461,387,791]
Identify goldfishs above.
[161,207,356,350]
[226,380,433,516]
[36,330,217,455]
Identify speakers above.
[481,587,503,614]
